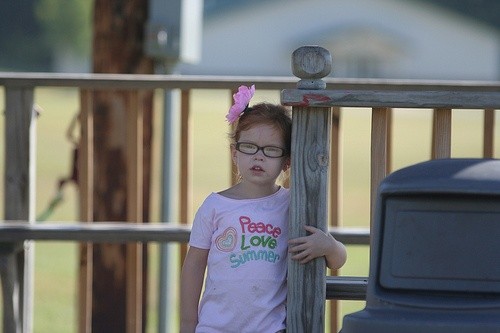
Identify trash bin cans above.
[338,158,500,333]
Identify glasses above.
[232,140,288,158]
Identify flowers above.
[225,84,255,127]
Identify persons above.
[181,84,348,333]
